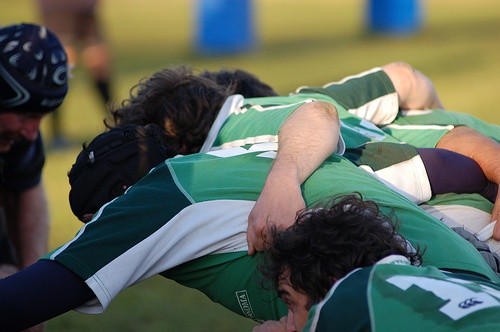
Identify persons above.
[0,60,500,332]
[37,0,117,150]
[0,23,69,269]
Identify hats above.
[0,24,68,113]
